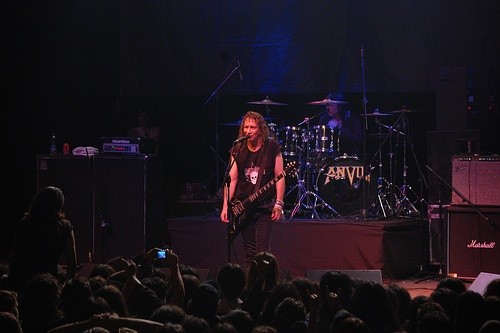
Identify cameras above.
[158,250,167,259]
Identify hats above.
[191,284,219,308]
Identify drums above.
[316,154,378,217]
[301,122,343,163]
[270,124,306,163]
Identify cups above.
[63,143,69,154]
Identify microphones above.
[361,49,363,68]
[489,221,498,228]
[237,60,243,80]
[353,182,359,189]
[234,135,249,143]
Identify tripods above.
[359,114,429,220]
[284,105,340,223]
[414,165,497,284]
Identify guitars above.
[227,160,300,239]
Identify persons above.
[7,186,77,277]
[0,247,500,333]
[320,93,361,137]
[220,111,286,287]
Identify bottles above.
[49,135,57,157]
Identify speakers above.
[35,154,162,266]
[445,152,500,298]
[306,270,383,285]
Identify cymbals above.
[384,109,421,114]
[245,99,289,106]
[305,98,352,106]
[358,112,395,116]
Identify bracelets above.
[274,199,284,214]
